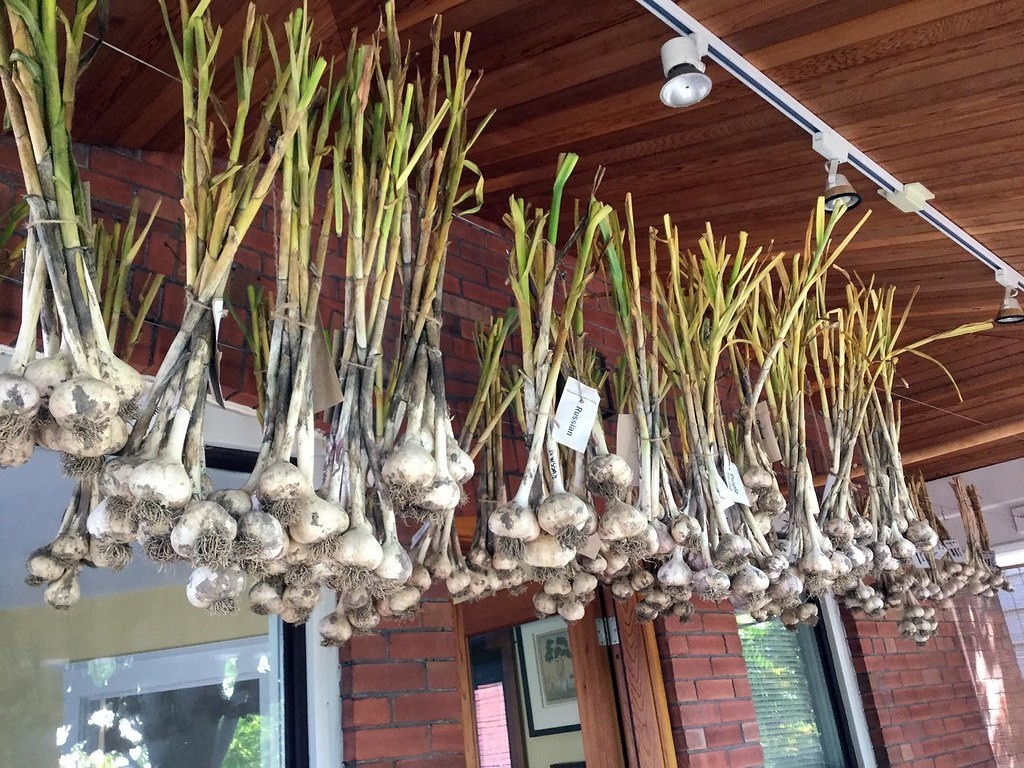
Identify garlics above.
[0,351,1016,648]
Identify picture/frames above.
[515,614,581,738]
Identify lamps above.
[994,268,1024,324]
[659,31,713,108]
[812,131,862,214]
